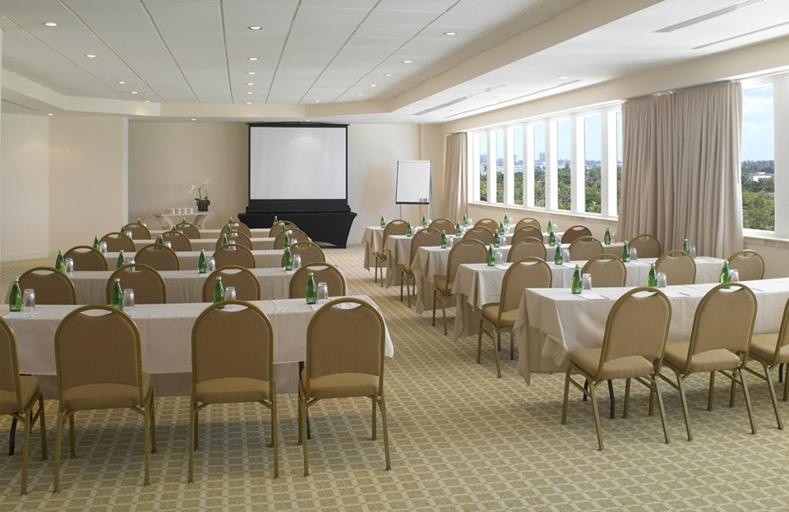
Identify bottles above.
[439,229,453,250]
[9,276,22,311]
[545,218,558,235]
[89,216,294,273]
[547,230,559,249]
[496,221,509,237]
[647,263,657,291]
[486,244,504,267]
[461,213,474,228]
[571,264,582,293]
[621,239,630,266]
[418,213,432,231]
[453,220,465,238]
[602,225,616,248]
[490,231,507,248]
[719,261,730,289]
[54,251,66,274]
[405,220,418,239]
[214,277,225,309]
[552,241,570,267]
[380,215,389,230]
[306,272,317,303]
[502,213,514,227]
[111,278,122,311]
[166,208,193,215]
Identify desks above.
[154,212,208,228]
[512,276,788,418]
[1,295,395,457]
[60,266,336,302]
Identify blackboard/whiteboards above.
[395,160,432,205]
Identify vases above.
[196,200,211,213]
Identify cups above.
[22,288,35,314]
[225,287,236,305]
[689,246,696,257]
[657,272,667,289]
[64,257,73,275]
[293,253,301,269]
[729,269,739,282]
[317,282,329,305]
[582,273,593,290]
[291,238,298,246]
[630,245,640,264]
[122,288,136,314]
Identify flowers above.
[187,177,210,199]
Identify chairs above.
[202,267,261,299]
[13,267,76,305]
[187,300,279,483]
[665,282,757,441]
[562,285,672,448]
[363,217,765,336]
[106,264,167,303]
[289,262,345,298]
[730,297,788,430]
[1,318,48,496]
[67,221,328,270]
[299,296,391,477]
[477,257,552,379]
[53,305,155,493]
